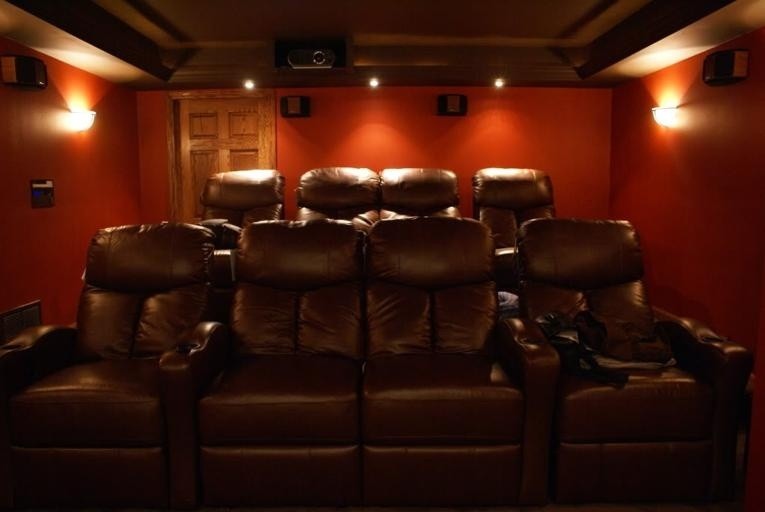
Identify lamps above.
[652,106,680,127]
[243,79,254,90]
[493,77,504,89]
[369,77,379,88]
[68,110,97,132]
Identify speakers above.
[701,49,749,85]
[437,93,470,116]
[281,94,312,118]
[2,53,49,90]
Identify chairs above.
[362,217,559,512]
[499,217,753,512]
[160,219,363,512]
[379,168,461,219]
[0,222,226,512]
[295,166,379,231]
[472,167,555,249]
[199,169,285,228]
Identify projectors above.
[285,44,339,70]
